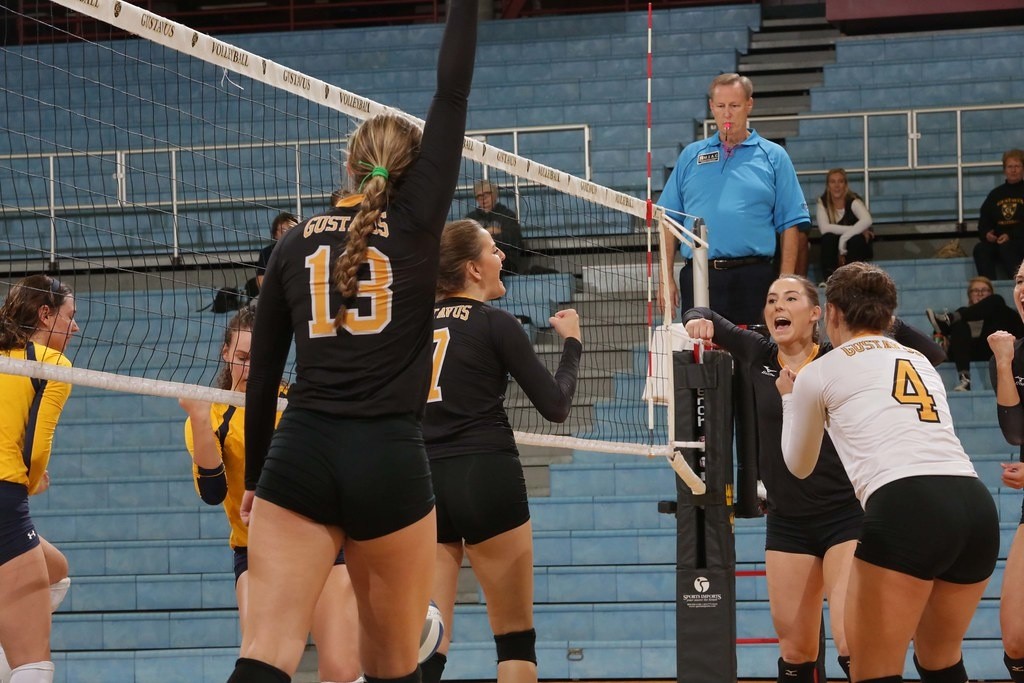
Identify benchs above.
[2,4,1024,683]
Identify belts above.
[687,256,771,270]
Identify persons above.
[420,218,582,683]
[986,263,1024,683]
[229,1,480,683]
[776,263,1000,683]
[0,274,79,683]
[815,169,874,282]
[177,307,364,683]
[924,276,1011,393]
[973,148,1024,278]
[466,180,525,276]
[258,212,299,288]
[656,73,811,494]
[682,272,865,683]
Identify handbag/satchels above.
[212,288,245,312]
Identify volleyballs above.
[416,598,444,664]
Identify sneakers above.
[954,374,971,391]
[927,307,952,335]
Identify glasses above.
[970,288,990,295]
